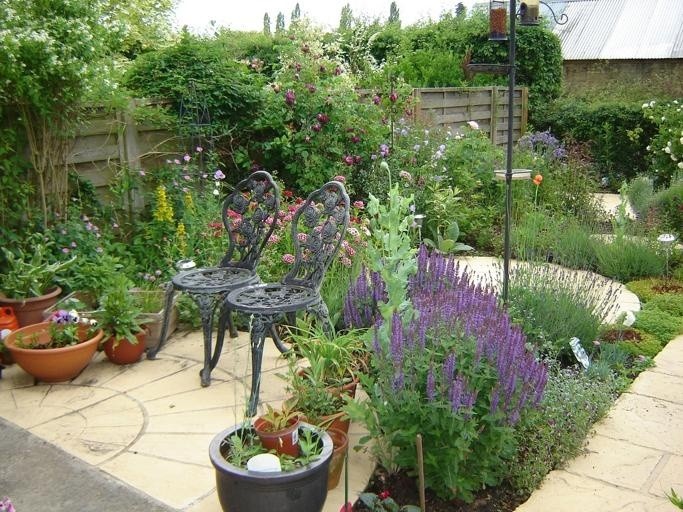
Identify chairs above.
[198,178,353,419]
[143,169,294,388]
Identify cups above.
[246,453,282,473]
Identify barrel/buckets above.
[0,306,20,332]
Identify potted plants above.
[0,238,183,385]
[204,310,373,512]
